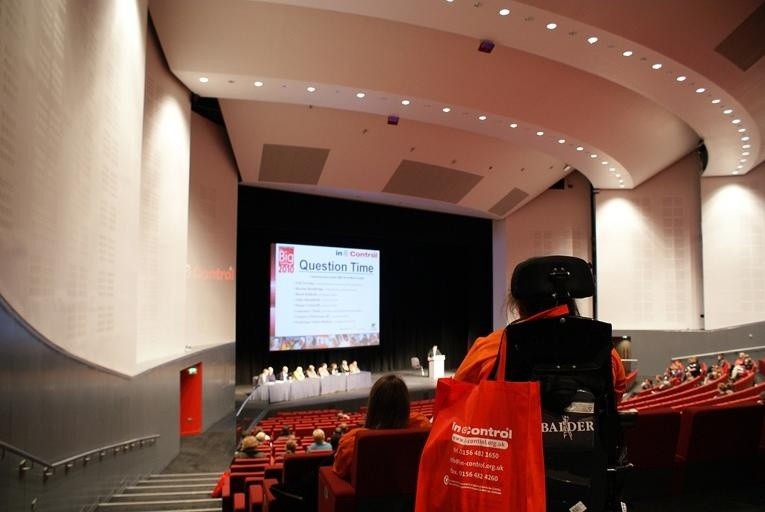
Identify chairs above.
[412,358,423,375]
[617,353,765,511]
[213,397,433,509]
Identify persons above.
[332,374,432,482]
[233,422,364,459]
[258,360,360,385]
[451,255,637,408]
[630,352,752,399]
[428,346,442,361]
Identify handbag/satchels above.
[413,330,548,512]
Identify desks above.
[252,371,372,403]
